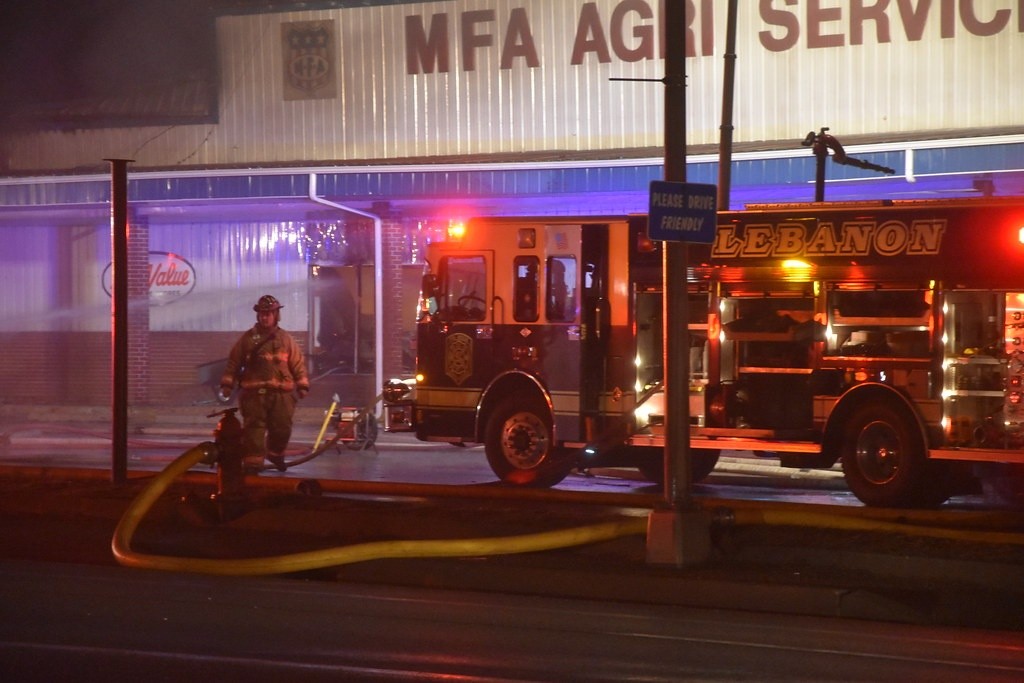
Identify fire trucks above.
[384,125,1023,510]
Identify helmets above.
[254,295,284,312]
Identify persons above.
[219,291,309,475]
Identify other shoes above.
[267,452,287,471]
